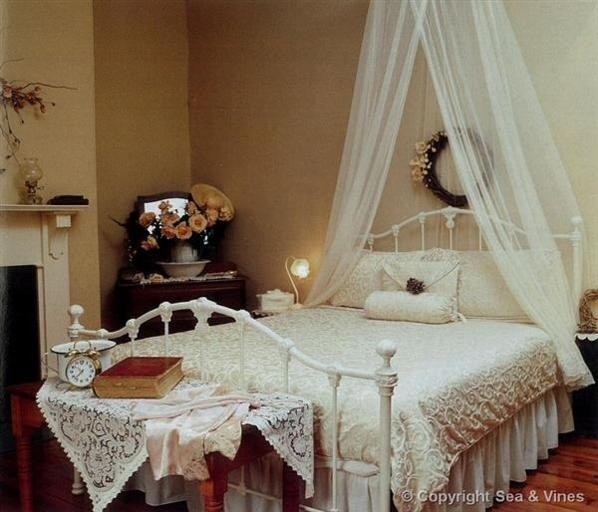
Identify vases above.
[157,245,212,278]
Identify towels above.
[133,381,257,485]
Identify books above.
[204,261,238,279]
[49,195,88,205]
[92,356,185,398]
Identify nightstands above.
[229,304,271,323]
[572,330,597,439]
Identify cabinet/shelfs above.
[116,272,250,337]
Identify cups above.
[41,338,118,384]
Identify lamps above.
[282,253,312,306]
[19,156,48,206]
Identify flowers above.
[0,57,81,175]
[406,125,494,207]
[102,183,239,264]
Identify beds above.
[67,209,580,512]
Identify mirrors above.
[138,191,199,240]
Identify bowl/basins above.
[157,260,211,279]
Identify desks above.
[0,376,315,512]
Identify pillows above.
[380,255,460,302]
[362,290,456,324]
[327,244,457,308]
[440,242,563,320]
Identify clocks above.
[61,335,103,393]
